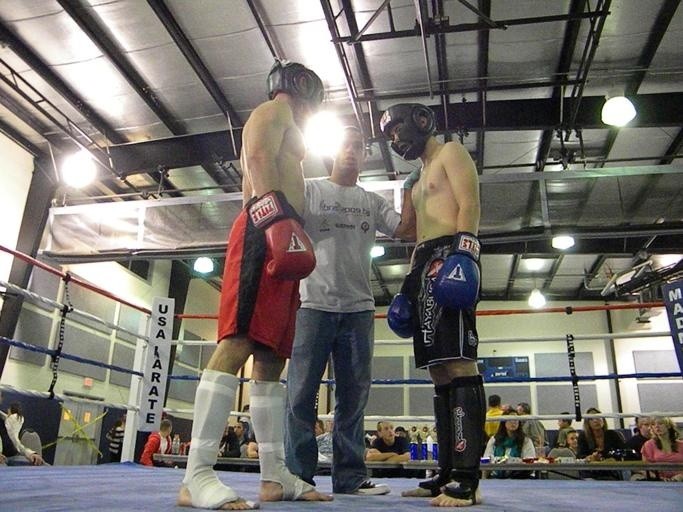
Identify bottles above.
[409,433,418,459]
[431,433,437,460]
[171,433,179,453]
[420,432,427,459]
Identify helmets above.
[380,103,439,159]
[267,58,324,111]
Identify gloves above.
[432,232,481,309]
[387,274,415,339]
[246,190,316,280]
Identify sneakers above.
[353,480,391,494]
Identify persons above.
[177,60,333,510]
[4,400,25,439]
[287,128,423,498]
[378,103,486,506]
[0,414,45,467]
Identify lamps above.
[601,90,638,128]
[527,273,547,308]
[193,257,215,274]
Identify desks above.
[401,458,682,480]
[150,451,400,476]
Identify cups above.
[520,452,534,464]
[479,458,489,464]
[545,453,554,464]
